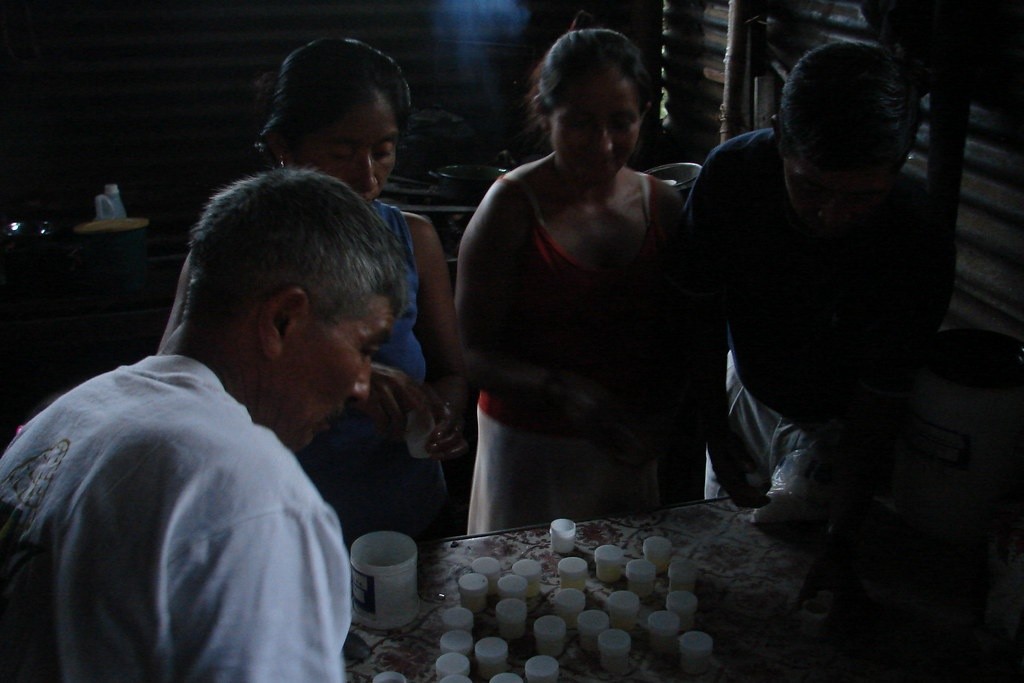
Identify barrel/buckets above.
[893,328,1024,542]
[350,532,420,630]
[644,162,703,208]
[437,165,505,206]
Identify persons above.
[667,42,957,512]
[452,28,686,537]
[0,167,407,682]
[159,35,468,549]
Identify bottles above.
[96,184,127,221]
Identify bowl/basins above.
[2,220,54,237]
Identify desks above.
[345,495,1024,683]
